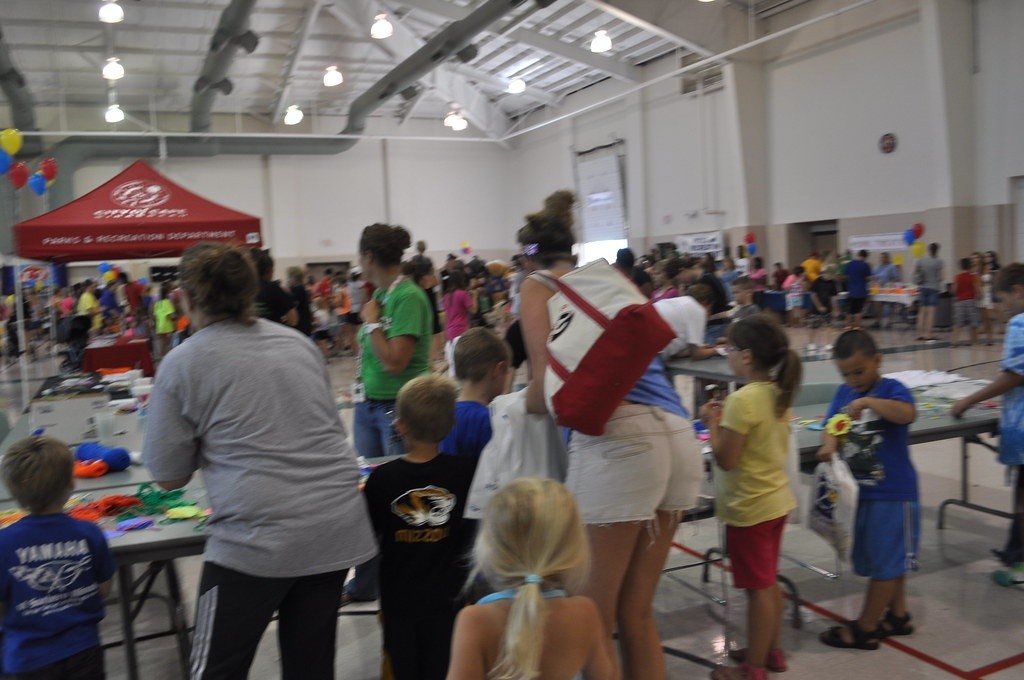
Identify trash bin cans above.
[935,292,951,328]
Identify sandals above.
[710,659,770,680]
[876,613,913,636]
[730,644,787,672]
[821,619,878,648]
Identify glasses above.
[723,344,741,353]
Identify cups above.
[131,377,155,416]
[96,412,117,447]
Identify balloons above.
[911,241,925,258]
[745,233,755,244]
[912,223,923,239]
[459,240,471,254]
[0,128,59,197]
[746,244,757,255]
[97,263,120,283]
[903,229,916,245]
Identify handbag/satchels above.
[525,258,678,439]
[786,429,803,527]
[807,455,860,559]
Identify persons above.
[813,330,920,652]
[697,316,804,680]
[141,243,379,680]
[774,239,1002,340]
[610,243,767,360]
[439,253,528,377]
[1,273,194,373]
[0,435,115,680]
[445,479,622,680]
[438,327,509,456]
[338,220,434,607]
[247,241,442,372]
[364,374,473,680]
[952,261,1024,590]
[516,189,706,680]
[504,319,529,395]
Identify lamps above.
[507,78,526,94]
[105,101,123,122]
[371,11,393,39]
[443,105,468,132]
[103,57,124,79]
[591,27,612,52]
[99,0,125,22]
[283,106,303,125]
[324,64,344,87]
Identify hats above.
[351,265,364,276]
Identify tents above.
[10,158,263,407]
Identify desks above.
[756,290,813,326]
[649,351,1024,630]
[831,282,921,329]
[1,331,209,680]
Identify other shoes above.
[854,326,865,330]
[806,343,816,350]
[339,585,379,606]
[916,334,941,341]
[992,568,1024,591]
[823,343,834,350]
[842,324,852,329]
[340,344,353,355]
